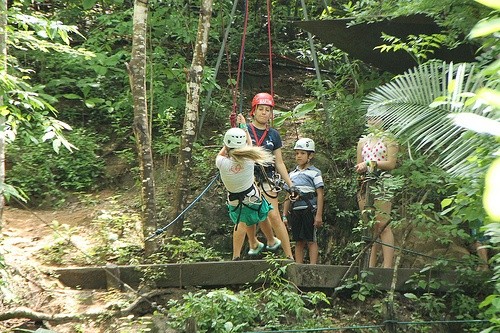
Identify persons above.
[474,241,490,277]
[233,93,300,264]
[356,110,394,269]
[215,127,281,256]
[282,137,324,264]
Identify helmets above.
[252,93,275,107]
[294,138,316,152]
[223,128,247,148]
[365,103,387,117]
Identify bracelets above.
[366,160,379,171]
[283,212,288,220]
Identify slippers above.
[266,236,281,250]
[247,242,264,255]
